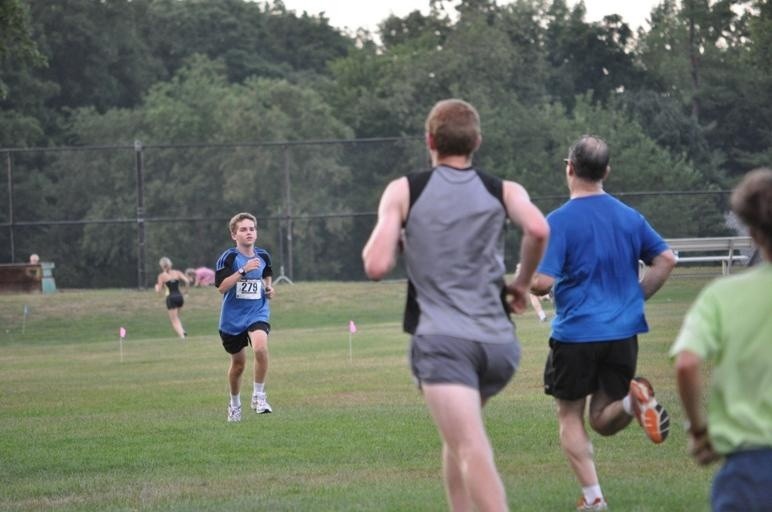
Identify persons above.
[154,257,189,338]
[25,254,42,280]
[185,267,215,288]
[668,166,772,512]
[530,136,675,512]
[362,98,551,512]
[215,212,275,422]
[514,262,551,322]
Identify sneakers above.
[250,392,272,414]
[629,376,670,444]
[576,496,608,512]
[227,401,242,422]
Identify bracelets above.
[685,425,709,441]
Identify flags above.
[349,321,356,333]
[120,326,126,337]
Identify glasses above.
[564,159,569,165]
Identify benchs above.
[0,264,43,294]
[639,236,753,281]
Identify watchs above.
[238,268,246,276]
